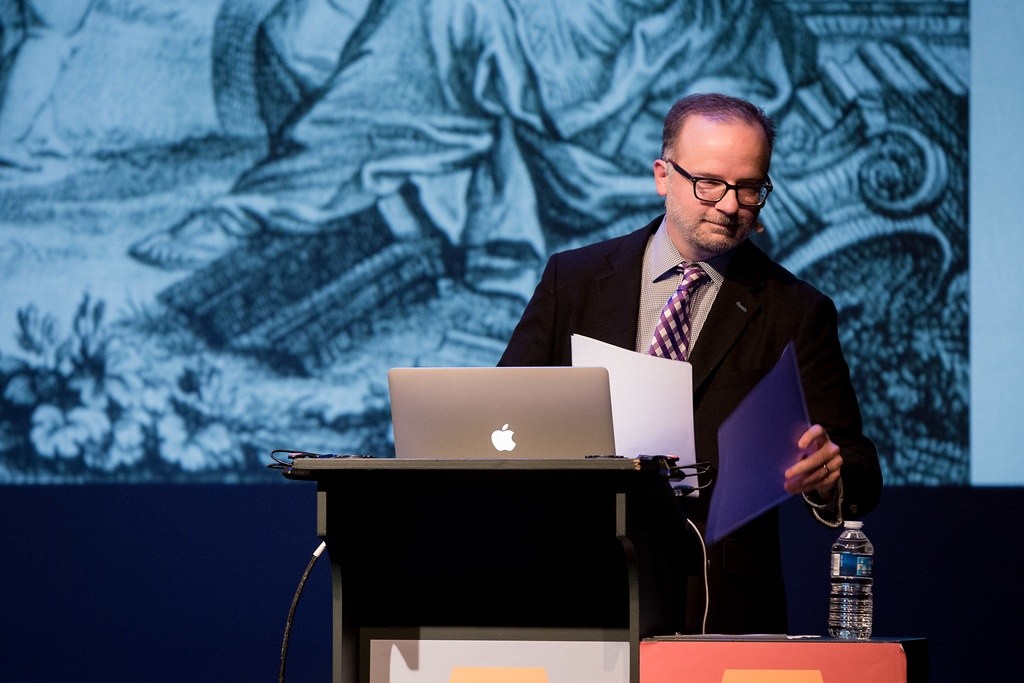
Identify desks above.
[292,454,644,683]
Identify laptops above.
[385,366,618,458]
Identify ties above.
[646,263,709,362]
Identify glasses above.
[669,160,773,208]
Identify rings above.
[823,464,830,474]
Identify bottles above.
[829,521,873,640]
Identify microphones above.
[755,223,765,233]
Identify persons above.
[495,93,885,633]
